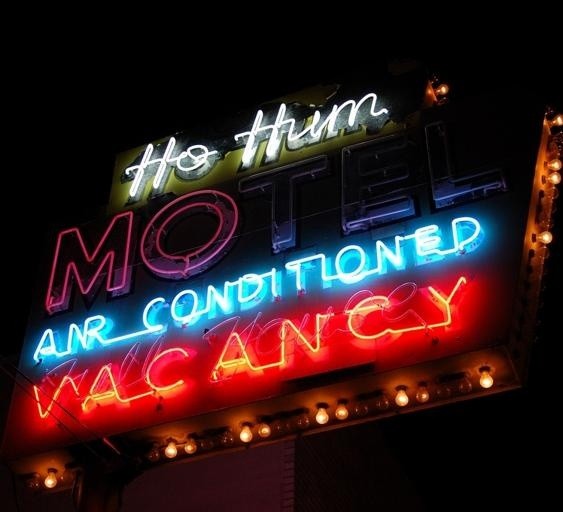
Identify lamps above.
[145,398,350,461]
[350,362,495,417]
[26,463,76,488]
[512,104,562,376]
